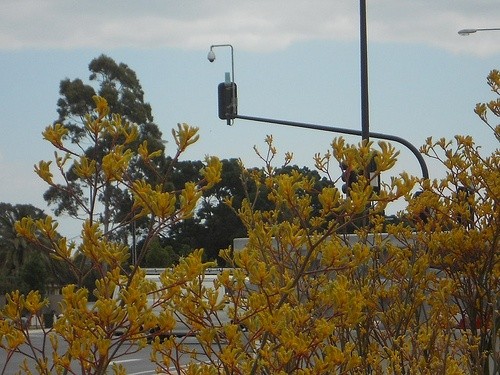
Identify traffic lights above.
[217,82,238,120]
